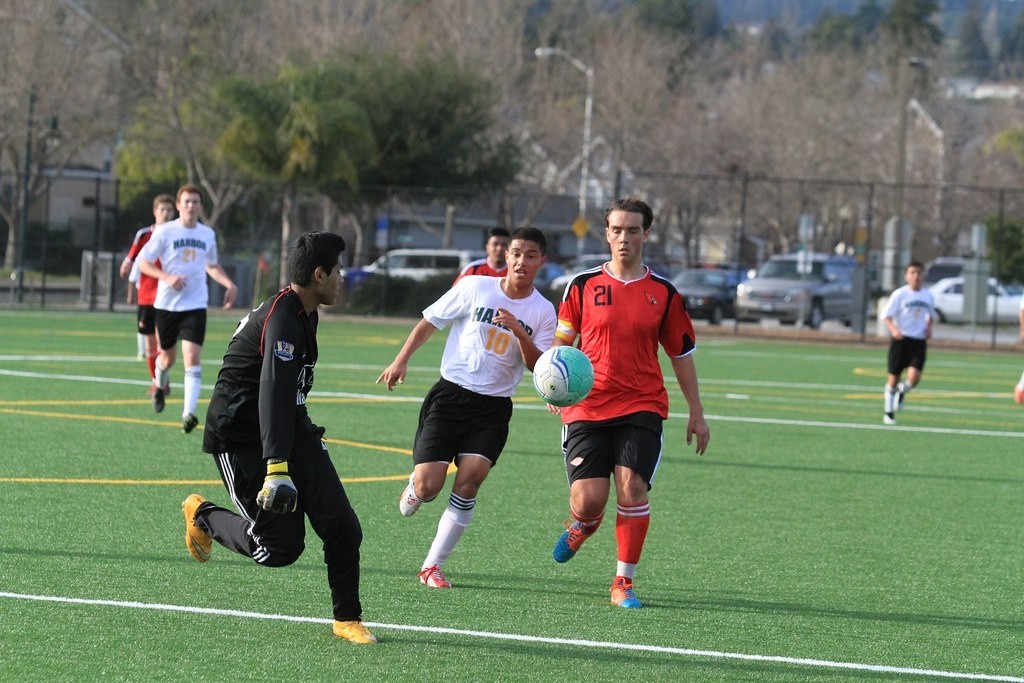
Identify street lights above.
[535,45,595,283]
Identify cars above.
[530,251,868,331]
[335,249,490,312]
[924,257,973,286]
[928,277,1024,323]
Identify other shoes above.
[136,354,146,361]
[895,383,905,410]
[883,414,896,425]
[149,381,170,395]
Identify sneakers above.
[609,576,644,609]
[553,517,603,563]
[334,620,378,644]
[181,414,198,433]
[183,494,213,562]
[150,384,164,413]
[399,484,421,517]
[418,564,452,589]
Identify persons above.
[547,199,710,609]
[134,182,238,433]
[452,226,509,288]
[880,262,935,425]
[375,229,557,587]
[182,232,377,644]
[119,194,177,397]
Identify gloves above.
[257,458,298,514]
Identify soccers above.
[532,345,594,407]
